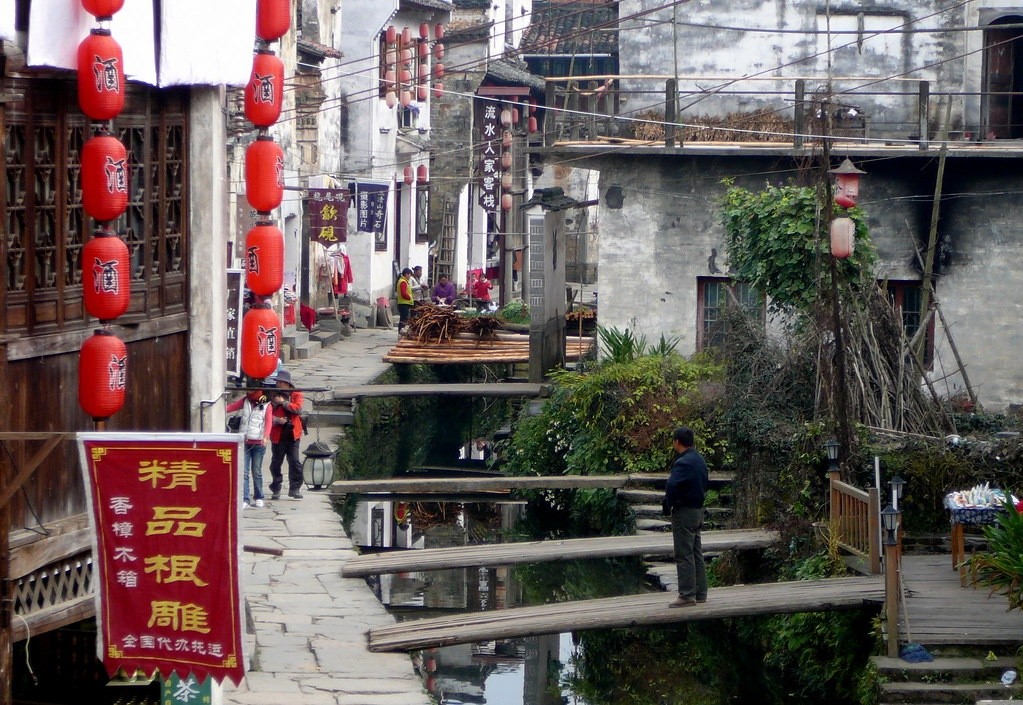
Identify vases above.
[566,318,595,331]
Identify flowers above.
[565,306,596,321]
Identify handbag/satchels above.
[226,417,241,433]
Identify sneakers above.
[696,596,706,603]
[669,597,696,608]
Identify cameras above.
[284,417,295,430]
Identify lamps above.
[887,473,907,511]
[820,436,840,472]
[225,384,335,490]
[880,502,900,545]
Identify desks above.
[944,489,1020,587]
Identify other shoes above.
[243,502,250,508]
[289,488,303,498]
[272,490,281,499]
[255,499,263,507]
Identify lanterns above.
[385,22,444,109]
[241,303,280,381]
[246,136,284,220]
[82,234,130,328]
[255,0,291,48]
[836,173,859,208]
[81,130,128,229]
[79,0,125,28]
[831,216,856,258]
[417,164,426,184]
[243,49,283,128]
[404,164,414,185]
[77,28,124,125]
[501,108,513,212]
[245,221,283,299]
[79,329,126,422]
[486,82,537,133]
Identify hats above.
[274,369,295,388]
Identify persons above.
[461,273,493,309]
[431,274,455,309]
[661,426,710,608]
[269,370,305,500]
[395,268,414,339]
[408,266,429,316]
[226,378,272,509]
